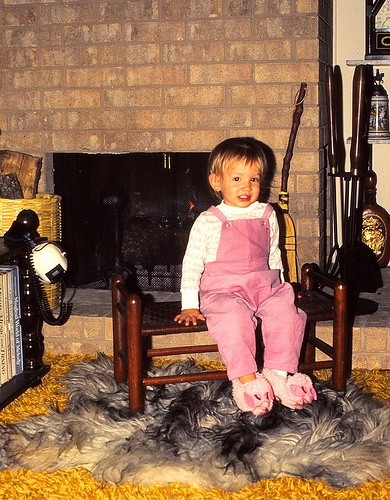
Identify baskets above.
[0,193,62,310]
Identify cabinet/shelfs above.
[346,60,390,144]
[0,208,53,413]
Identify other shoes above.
[232,372,274,416]
[262,368,318,410]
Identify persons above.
[174,138,317,416]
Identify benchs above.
[111,263,346,414]
